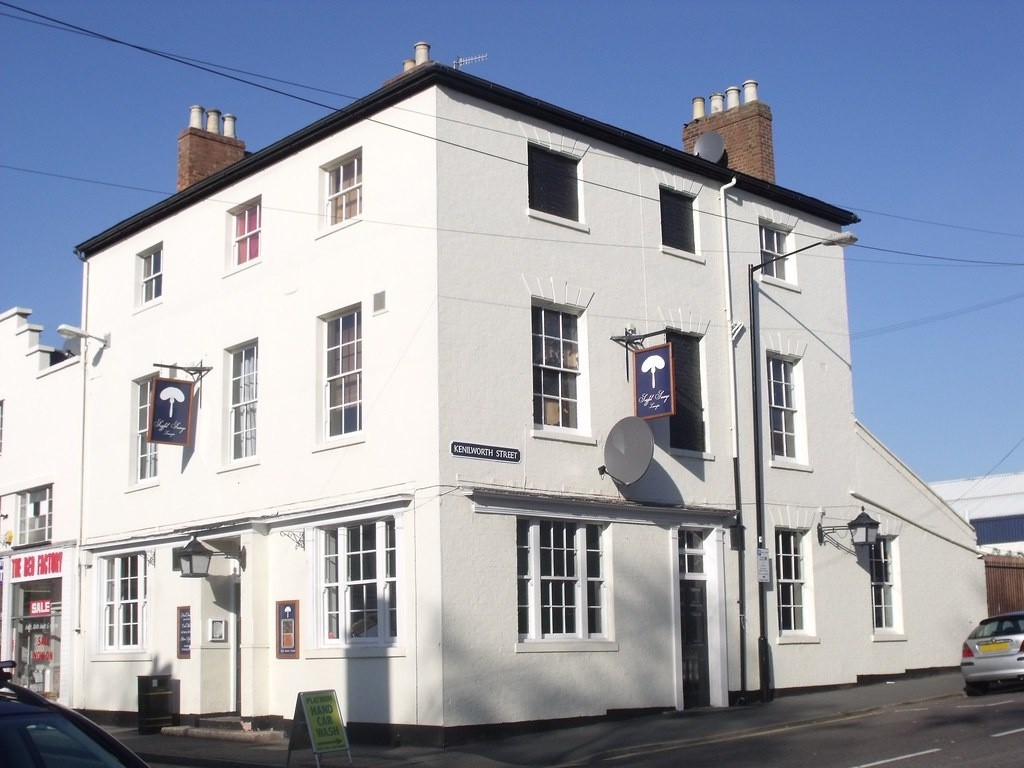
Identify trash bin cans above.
[136,675,172,735]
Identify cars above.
[1,660,151,768]
[961,610,1024,695]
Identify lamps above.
[176,532,248,578]
[818,505,880,548]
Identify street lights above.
[748,230,858,702]
